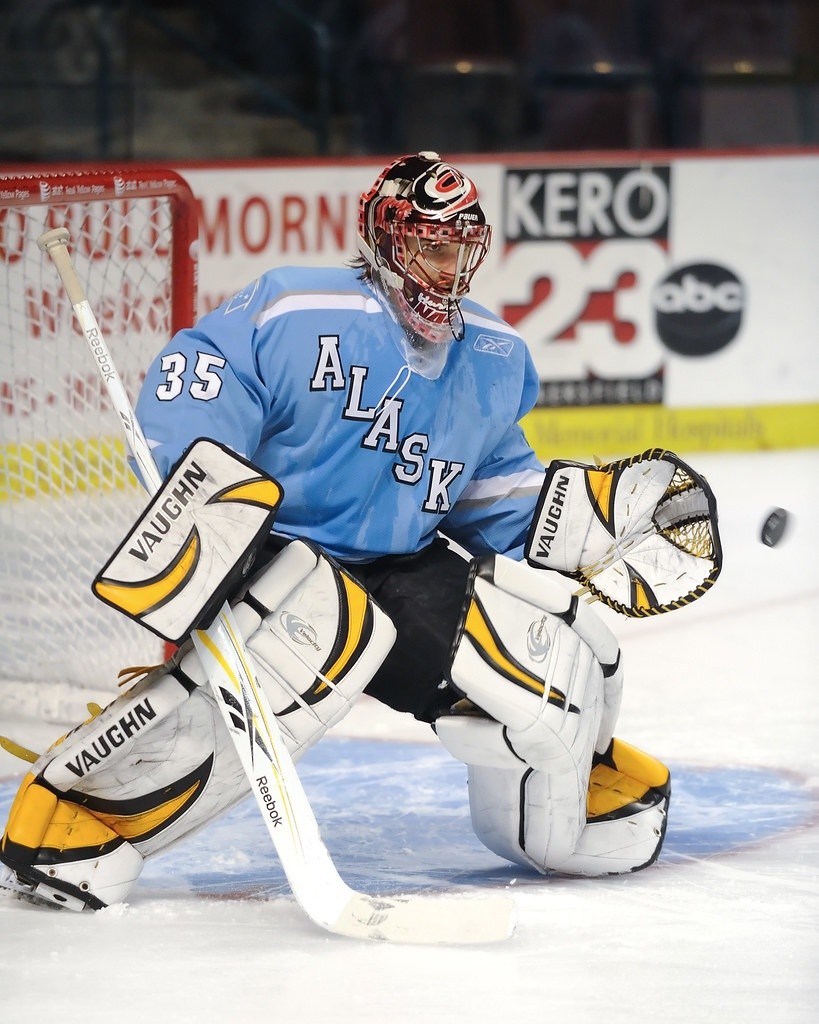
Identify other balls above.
[760,507,789,550]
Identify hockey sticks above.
[36,225,517,949]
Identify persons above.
[0,153,724,912]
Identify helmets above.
[355,149,492,343]
